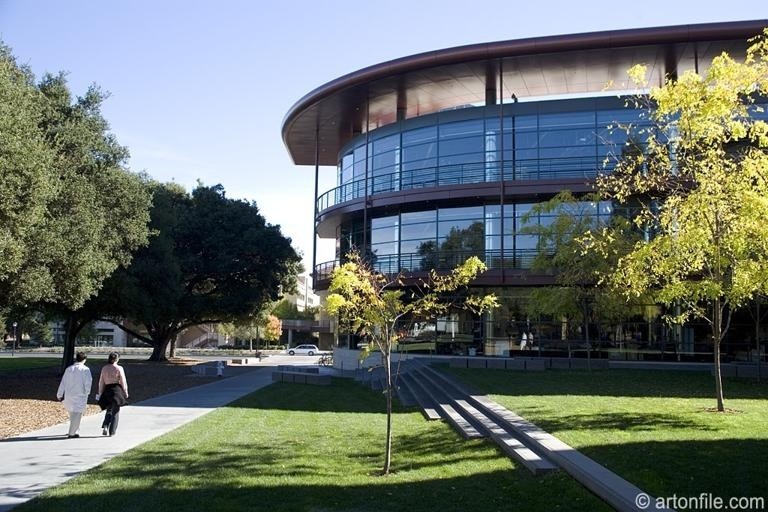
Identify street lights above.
[12,322,18,357]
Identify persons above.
[520,331,527,350]
[98,351,128,437]
[528,332,534,350]
[56,352,92,438]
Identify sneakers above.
[69,435,78,438]
[103,427,107,435]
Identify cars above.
[287,345,319,355]
